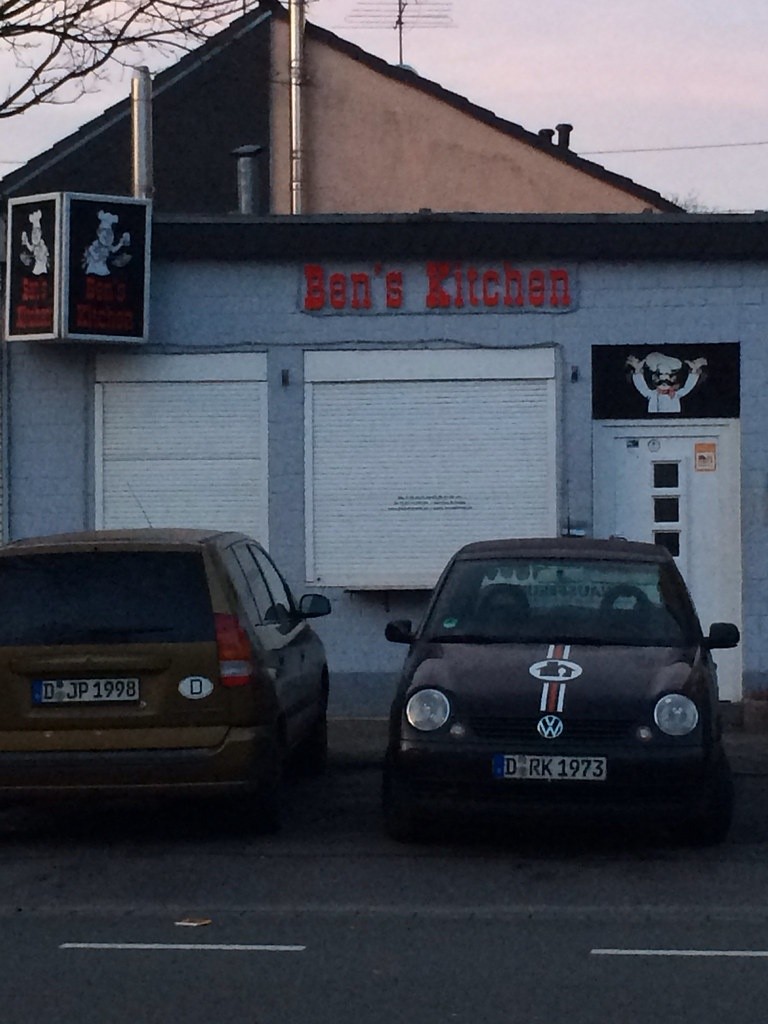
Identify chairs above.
[475,582,530,620]
[601,583,648,611]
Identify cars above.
[379,534,743,849]
[0,527,333,806]
